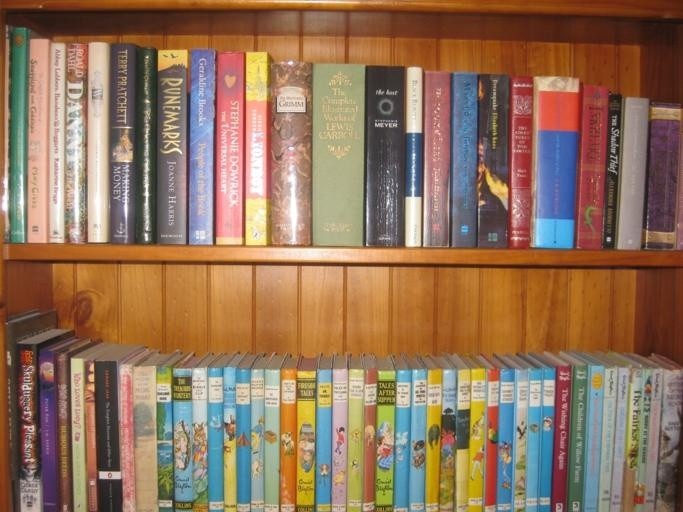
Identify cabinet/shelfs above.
[0,0,681,512]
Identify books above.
[1,24,682,252]
[0,308,683,512]
